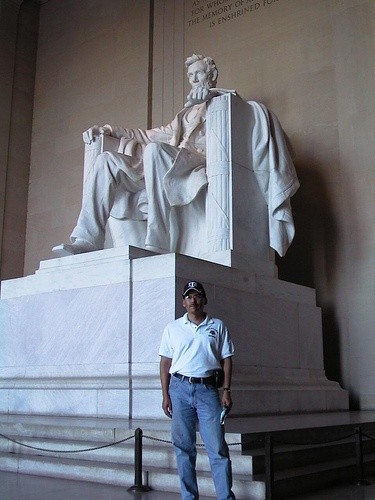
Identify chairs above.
[83,93,278,278]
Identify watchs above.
[224,387,232,392]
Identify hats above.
[182,280,207,296]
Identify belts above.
[173,373,214,385]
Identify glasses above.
[184,294,203,299]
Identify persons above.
[156,281,237,500]
[52,54,240,252]
